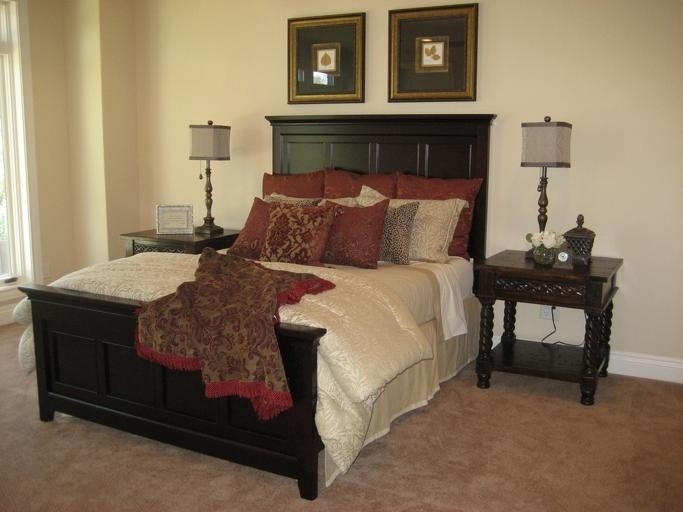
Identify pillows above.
[225,168,484,268]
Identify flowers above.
[525,228,565,253]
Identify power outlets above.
[539,303,560,321]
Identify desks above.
[120,223,241,259]
[470,247,624,407]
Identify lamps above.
[186,119,232,236]
[520,116,573,261]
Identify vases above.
[530,245,557,269]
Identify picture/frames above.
[285,10,367,106]
[387,4,479,104]
[155,202,194,237]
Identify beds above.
[16,111,500,502]
